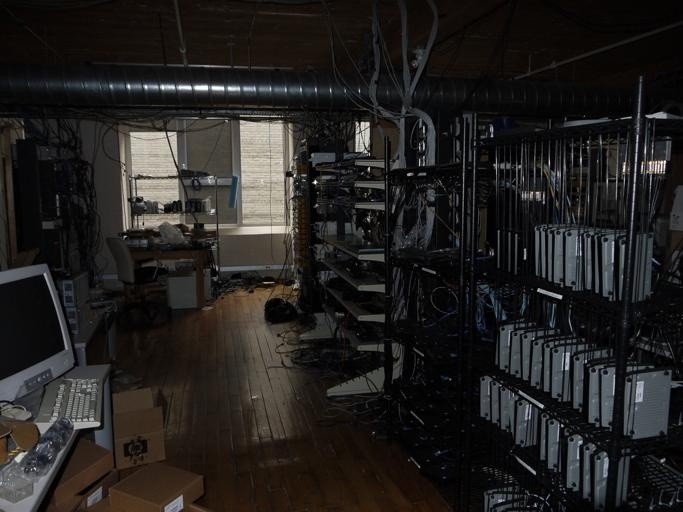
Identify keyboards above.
[32,375,104,429]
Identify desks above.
[0,364,114,512]
[126,240,211,306]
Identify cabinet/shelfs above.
[129,175,219,279]
[316,160,402,359]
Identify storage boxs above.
[38,388,212,512]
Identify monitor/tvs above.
[0,263,76,422]
